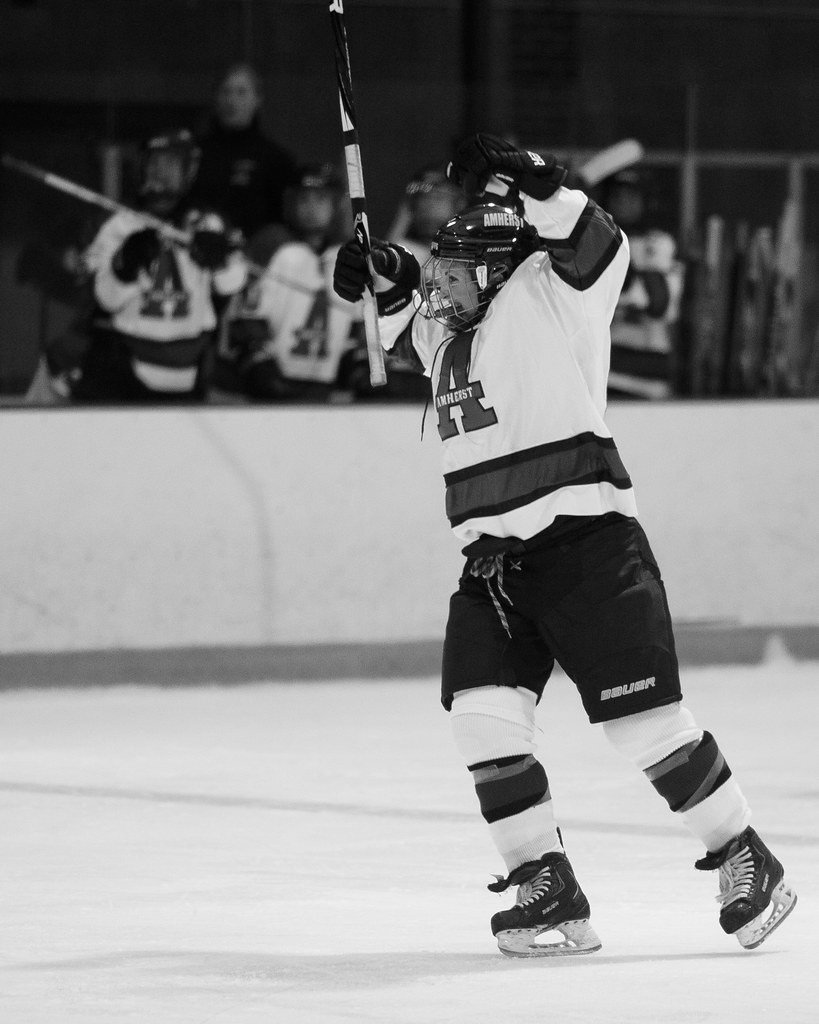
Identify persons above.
[8,55,701,405]
[330,128,801,957]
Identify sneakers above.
[489,858,602,955]
[694,827,798,948]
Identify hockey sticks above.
[3,154,353,310]
[322,0,390,388]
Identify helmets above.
[421,203,535,331]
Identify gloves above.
[444,133,566,208]
[332,238,422,317]
